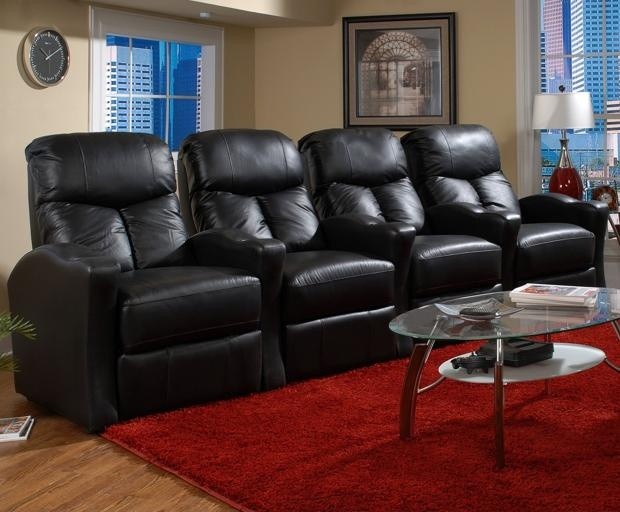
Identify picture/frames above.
[342,11,458,132]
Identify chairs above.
[4,131,280,436]
[297,128,516,355]
[400,122,610,293]
[176,129,411,392]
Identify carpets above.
[98,312,620,512]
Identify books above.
[0,415,31,438]
[508,282,600,309]
[0,418,34,443]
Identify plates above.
[433,296,524,319]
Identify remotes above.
[460,307,500,316]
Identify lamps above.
[530,90,595,201]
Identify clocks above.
[591,184,617,212]
[21,26,73,89]
